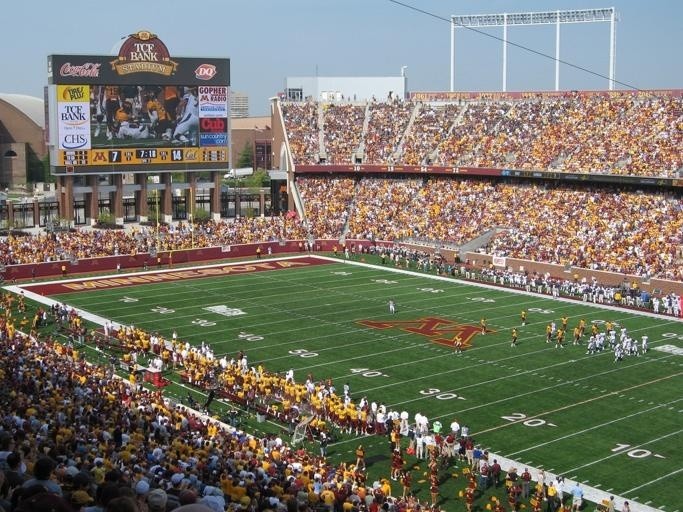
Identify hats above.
[71,490,95,504]
[179,486,198,505]
[135,480,150,495]
[13,492,72,512]
[171,473,185,485]
[147,488,168,510]
[64,466,81,480]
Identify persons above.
[0,217,269,286]
[269,91,683,319]
[94,85,199,146]
[1,290,649,512]
